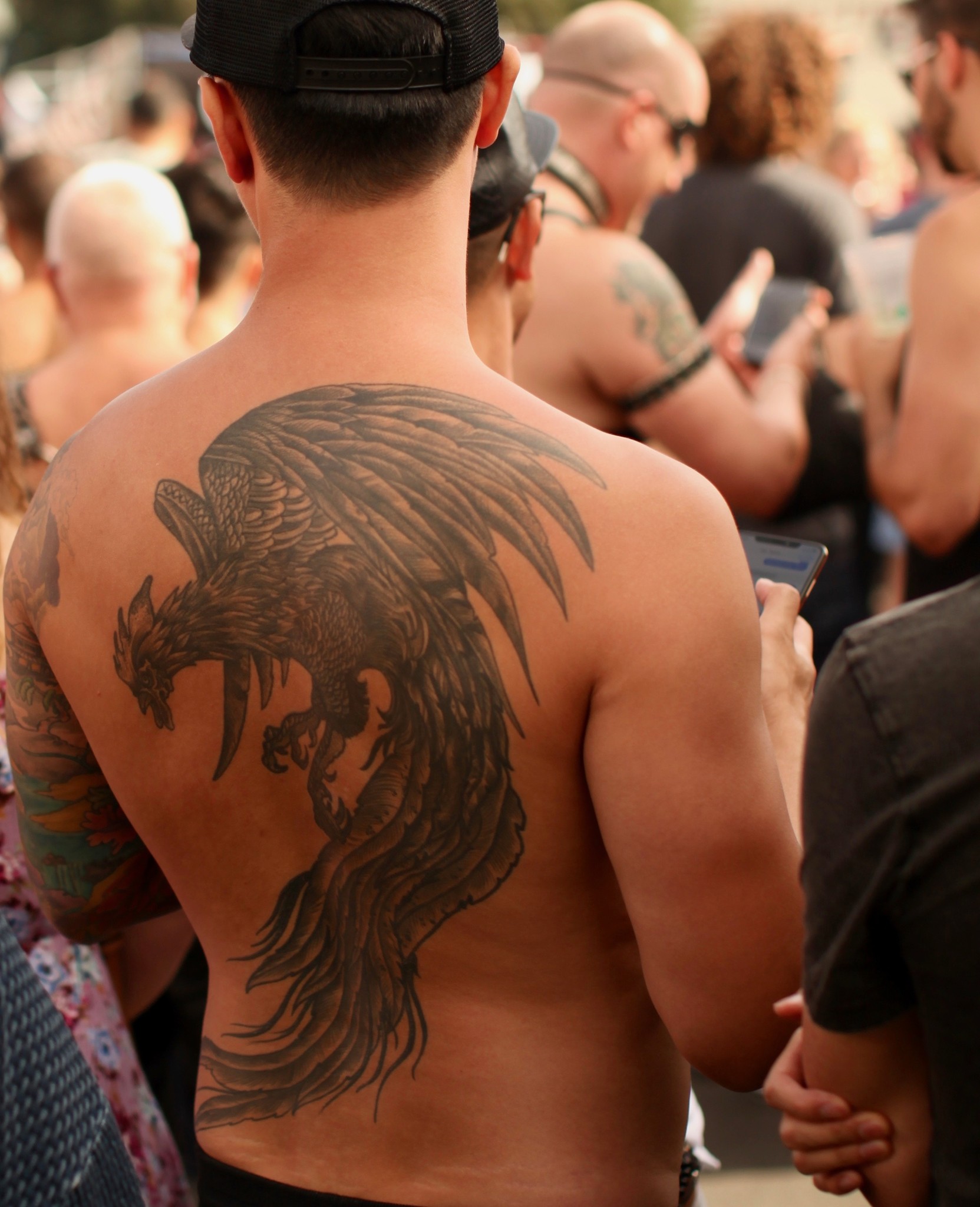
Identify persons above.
[0,0,811,1207]
[0,0,980,1207]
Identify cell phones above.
[740,278,813,364]
[740,530,830,619]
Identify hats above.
[181,0,506,86]
[469,88,557,239]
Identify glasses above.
[127,90,170,125]
[497,189,547,263]
[895,38,940,94]
[662,115,704,157]
